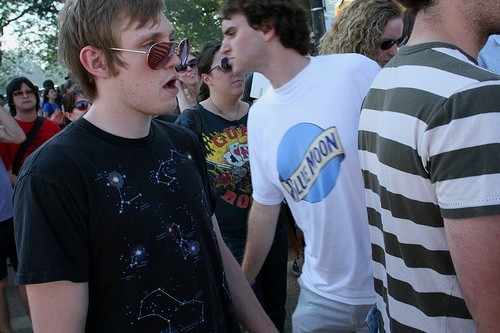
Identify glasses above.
[207,57,232,74]
[14,89,34,96]
[73,100,93,111]
[380,35,408,51]
[177,58,198,72]
[107,41,190,70]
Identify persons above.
[0,0,500,333]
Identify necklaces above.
[210,97,240,120]
